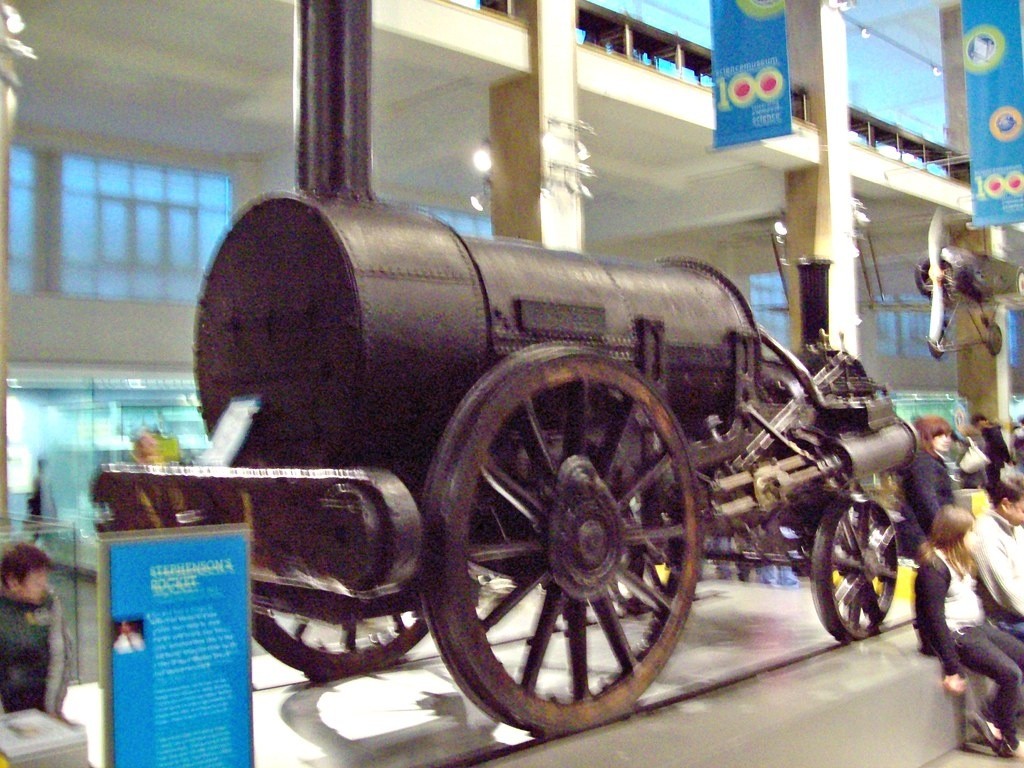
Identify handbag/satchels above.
[1000,463,1024,492]
[959,436,991,473]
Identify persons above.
[755,564,800,586]
[714,560,751,581]
[912,504,1024,758]
[971,414,1024,481]
[893,414,956,558]
[26,458,60,543]
[0,543,67,717]
[113,622,143,654]
[968,473,1024,618]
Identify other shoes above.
[1004,740,1023,756]
